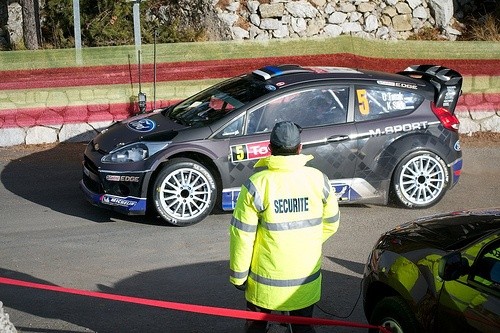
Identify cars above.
[80,63,463,228]
[362,206,500,333]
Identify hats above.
[270,117,302,150]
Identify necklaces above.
[227,120,342,333]
